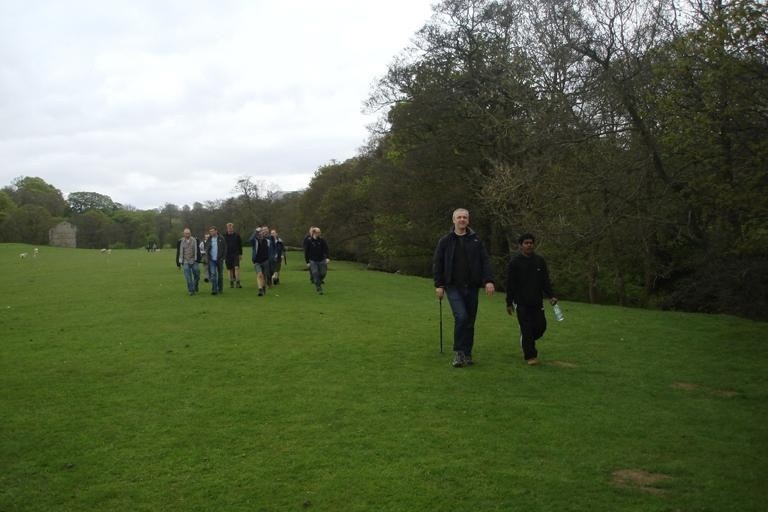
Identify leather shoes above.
[210,288,224,295]
[451,351,473,367]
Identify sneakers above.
[527,356,539,365]
[187,290,200,295]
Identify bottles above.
[553,304,564,322]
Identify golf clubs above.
[439,298,443,355]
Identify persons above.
[303,226,330,295]
[506,233,558,365]
[249,224,287,296]
[432,208,495,367]
[176,223,243,296]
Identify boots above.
[257,277,279,297]
[230,279,243,289]
[315,280,325,296]
